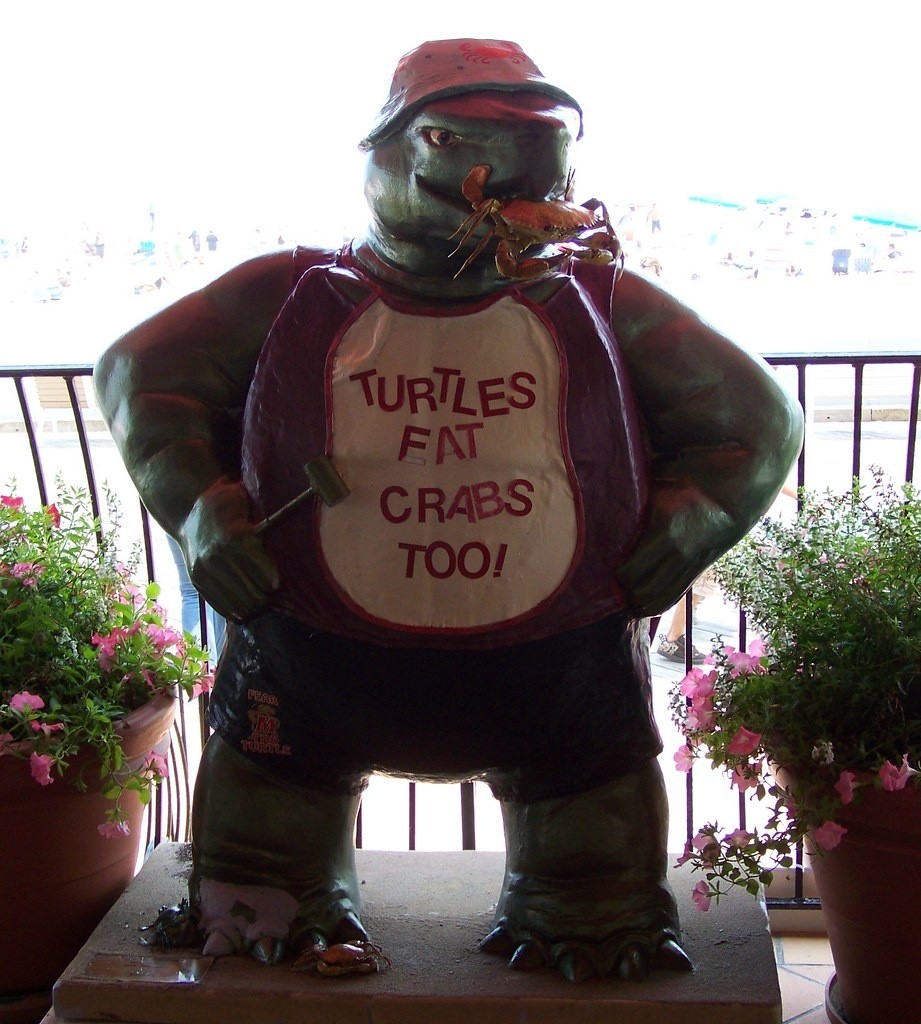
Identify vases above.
[762,743,921,1024]
[0,683,175,1024]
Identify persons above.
[658,485,796,663]
[632,203,904,281]
[1,208,217,303]
[165,535,227,668]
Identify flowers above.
[0,466,215,841]
[668,465,921,914]
